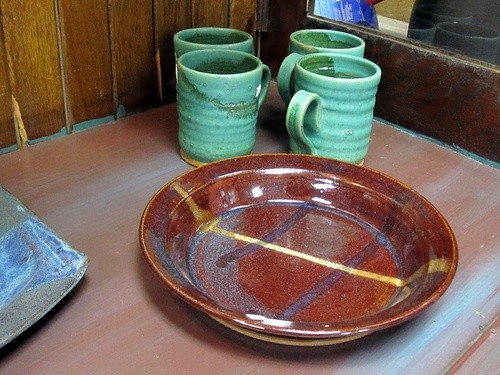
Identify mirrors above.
[307,0,500,67]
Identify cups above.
[285,53,382,165]
[175,49,271,166]
[277,29,366,105]
[173,27,254,62]
[408,4,499,65]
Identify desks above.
[0,80,500,375]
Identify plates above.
[139,153,459,346]
[1,185,88,349]
